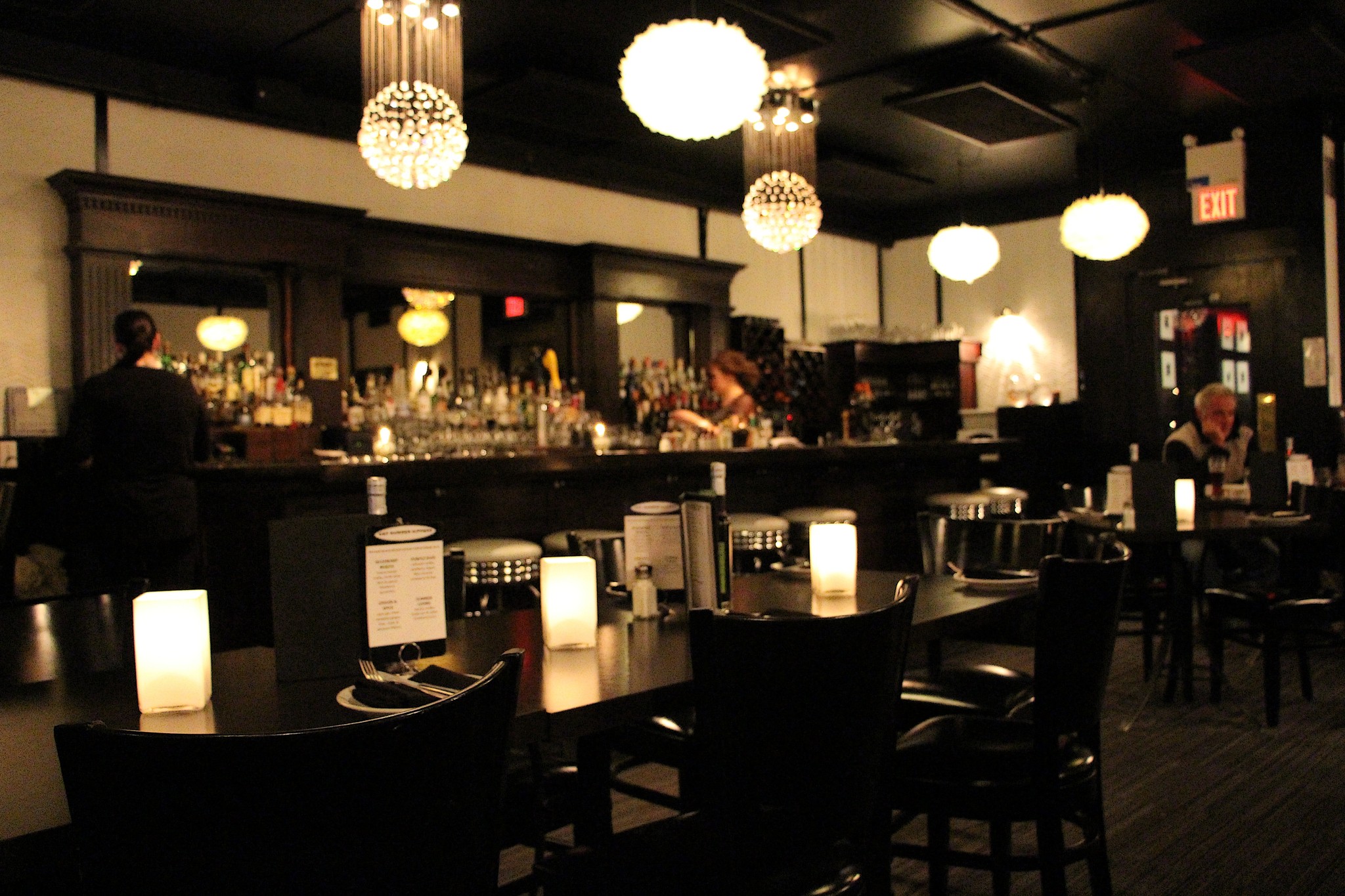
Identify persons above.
[1160,381,1261,509]
[669,349,764,435]
[68,311,222,468]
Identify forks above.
[358,658,385,682]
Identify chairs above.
[2,444,1345,896]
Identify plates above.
[336,670,488,714]
[952,567,1038,590]
[769,555,811,577]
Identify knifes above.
[377,668,454,698]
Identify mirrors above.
[336,282,492,397]
[127,267,276,383]
[612,300,710,396]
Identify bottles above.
[698,460,735,616]
[153,342,788,449]
[633,564,658,619]
[363,474,397,546]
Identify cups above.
[1207,456,1226,496]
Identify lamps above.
[739,65,826,258]
[611,0,772,143]
[1052,76,1153,263]
[926,159,1003,285]
[355,0,476,189]
[188,285,250,353]
[392,286,456,348]
